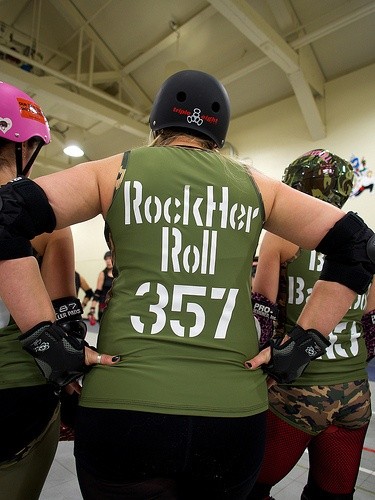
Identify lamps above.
[62,124,87,158]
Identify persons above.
[74,271,94,314]
[0,70,375,500]
[88,251,116,325]
[0,81,86,500]
[252,148,375,500]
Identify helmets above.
[281,149,354,209]
[0,81,51,145]
[148,69,231,149]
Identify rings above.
[97,353,102,364]
